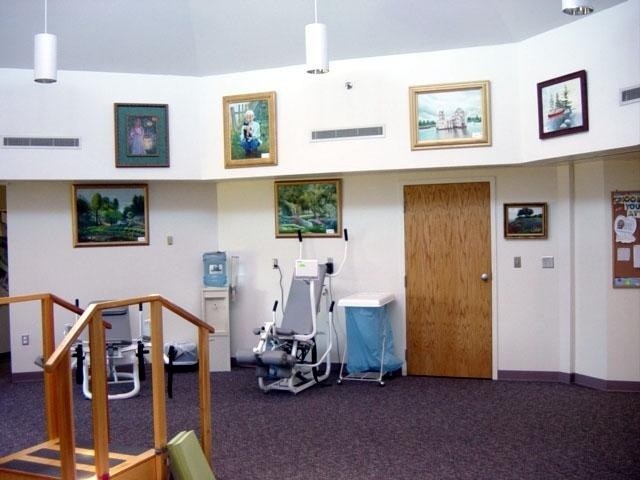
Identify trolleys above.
[337,293,403,387]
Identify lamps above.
[562,0,593,18]
[305,0,330,74]
[33,0,58,83]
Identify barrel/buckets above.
[203,251,227,287]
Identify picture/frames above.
[70,183,150,247]
[408,79,494,152]
[114,102,166,171]
[535,68,589,140]
[222,90,276,168]
[503,202,547,238]
[274,178,341,239]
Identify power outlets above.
[513,256,522,267]
[167,236,174,247]
[22,334,29,346]
[272,259,277,269]
